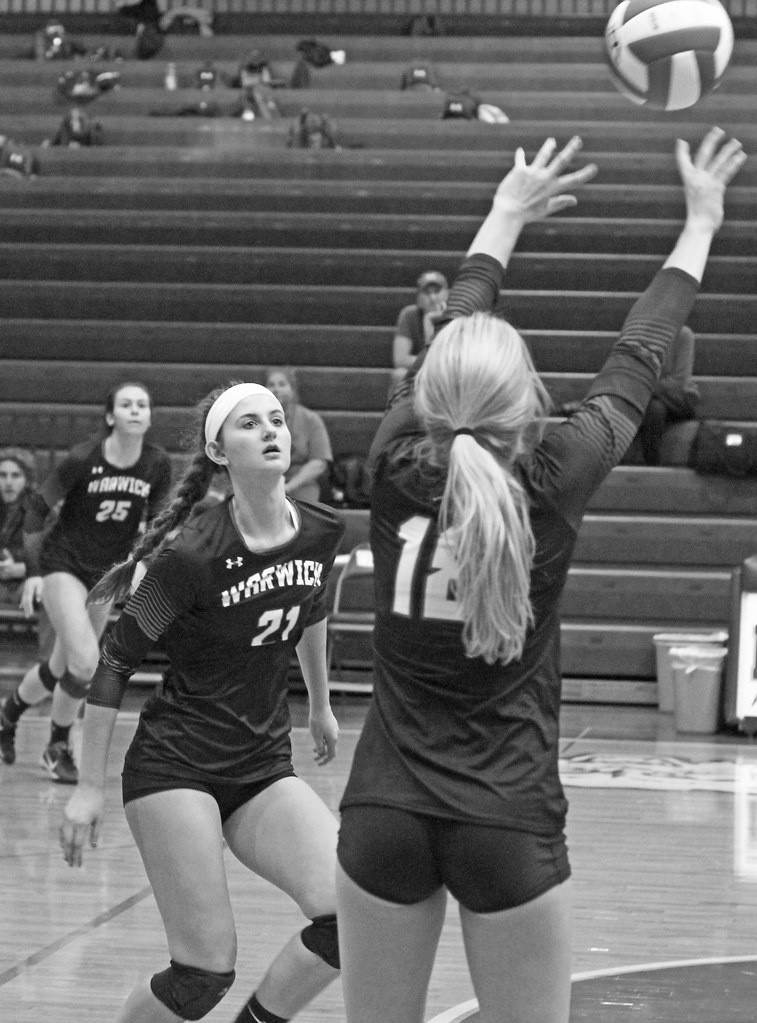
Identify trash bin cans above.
[651,630,729,712]
[667,646,728,735]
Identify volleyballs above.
[602,0,734,111]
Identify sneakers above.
[0,700,18,764]
[39,744,80,785]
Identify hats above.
[416,270,448,290]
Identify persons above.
[59,383,342,1023]
[333,127,747,1023]
[0,270,699,783]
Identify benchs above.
[0,28,757,684]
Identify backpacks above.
[41,108,109,149]
[238,84,283,120]
[442,97,471,119]
[399,63,442,94]
[284,107,341,151]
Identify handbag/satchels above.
[321,452,369,509]
[0,138,41,181]
[689,420,757,478]
